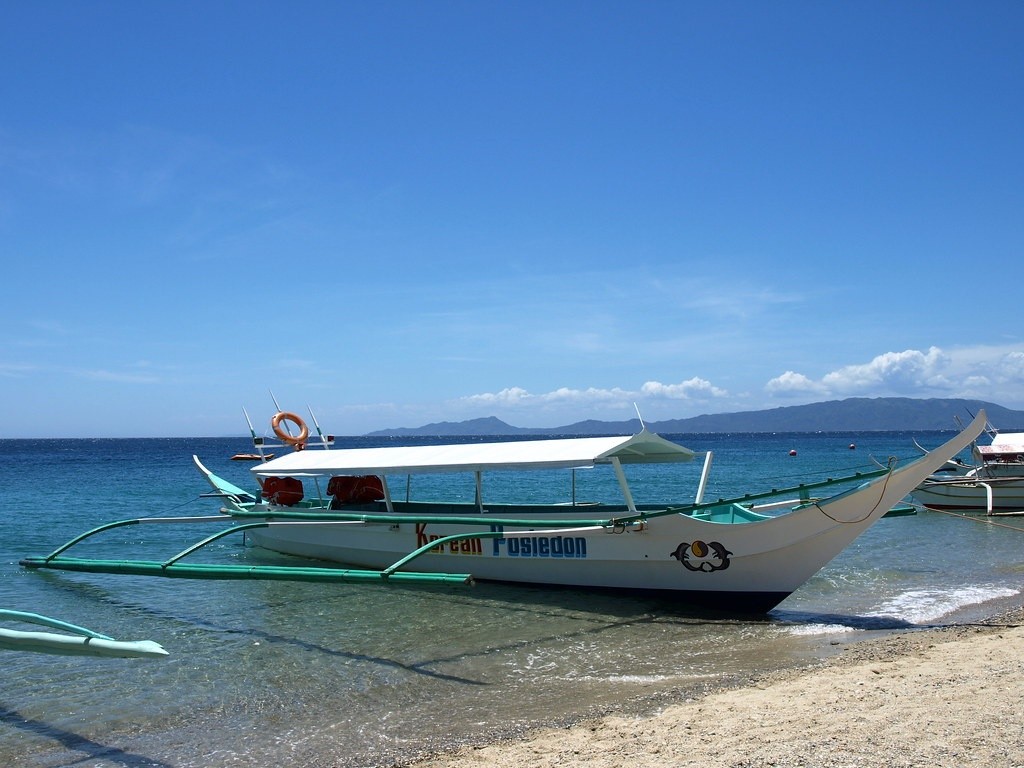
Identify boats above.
[944,414,945,445]
[20,390,987,621]
[868,415,1024,509]
[230,453,275,460]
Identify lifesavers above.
[271,412,310,446]
